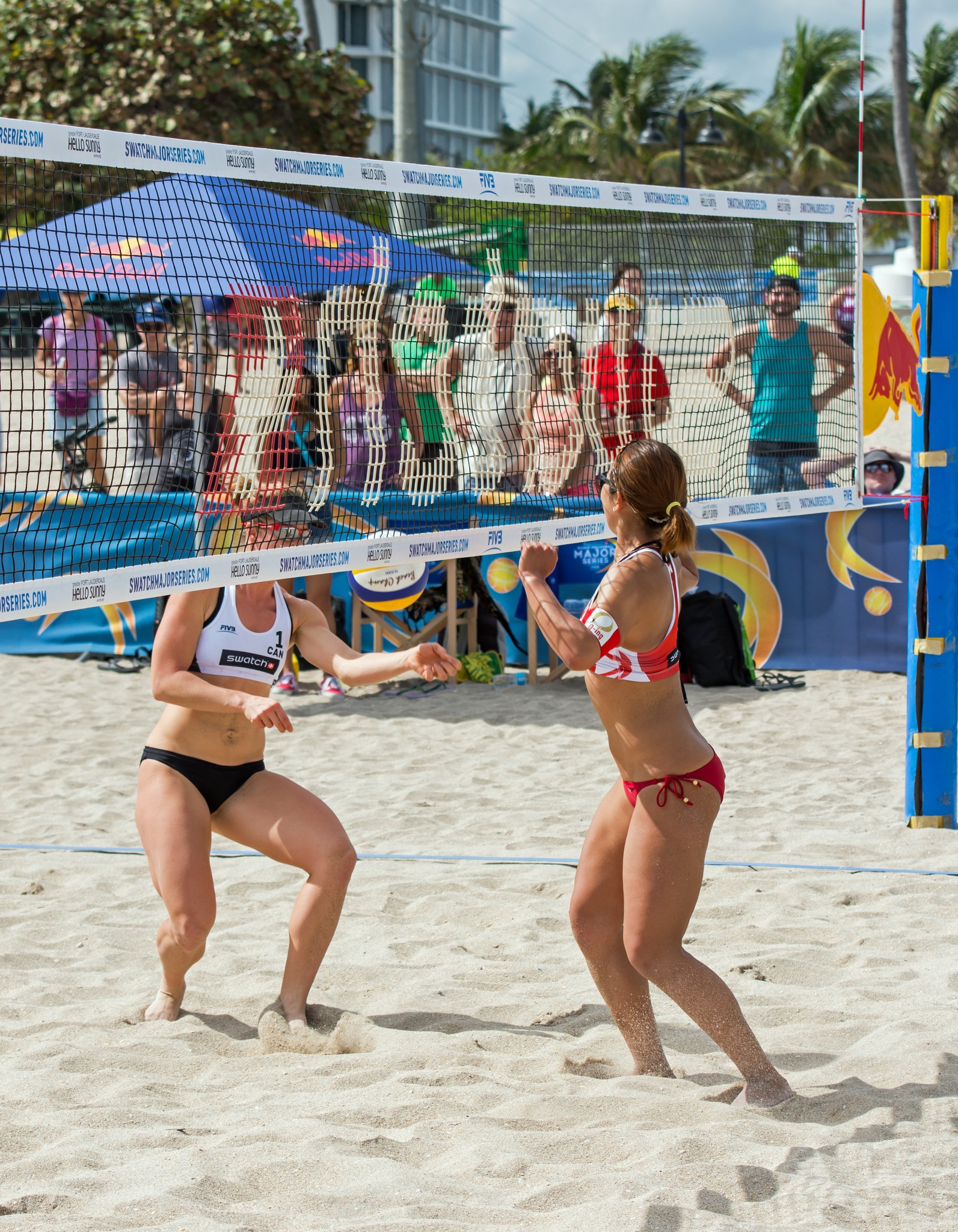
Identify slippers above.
[385,679,456,700]
[754,671,806,691]
[97,646,152,673]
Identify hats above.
[136,301,170,325]
[786,245,804,257]
[294,292,327,307]
[851,450,905,491]
[603,294,639,311]
[483,285,525,304]
[241,494,329,528]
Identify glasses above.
[241,521,313,544]
[181,354,212,365]
[599,471,636,514]
[543,350,570,360]
[356,341,386,350]
[863,463,894,473]
[139,322,164,331]
[487,303,516,313]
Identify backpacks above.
[681,590,759,688]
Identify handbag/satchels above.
[56,391,91,416]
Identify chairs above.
[529,507,605,687]
[353,515,480,686]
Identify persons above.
[769,244,804,280]
[135,489,462,1037]
[35,291,122,493]
[515,439,793,1112]
[827,270,854,384]
[706,275,855,494]
[233,350,344,698]
[801,446,912,497]
[115,262,674,499]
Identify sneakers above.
[272,671,299,695]
[320,675,346,700]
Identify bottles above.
[494,672,529,690]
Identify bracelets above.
[634,418,640,433]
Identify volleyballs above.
[347,528,430,613]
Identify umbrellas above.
[1,173,483,557]
